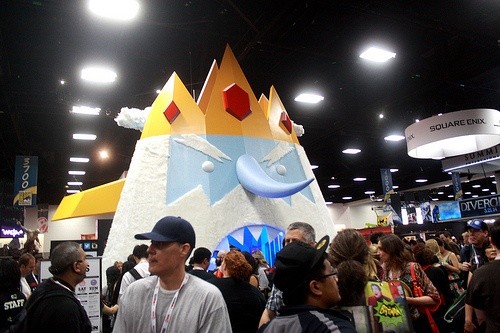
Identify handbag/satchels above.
[409,262,443,315]
[447,272,466,300]
[363,263,414,333]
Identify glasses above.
[323,268,339,280]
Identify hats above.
[255,250,264,259]
[133,215,196,245]
[466,219,489,232]
[32,253,42,259]
[271,235,330,293]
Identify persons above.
[15,242,92,333]
[259,222,316,328]
[258,234,357,333]
[329,220,500,333]
[0,253,40,333]
[17,221,40,253]
[185,247,270,333]
[368,284,391,333]
[102,244,152,333]
[112,216,232,333]
